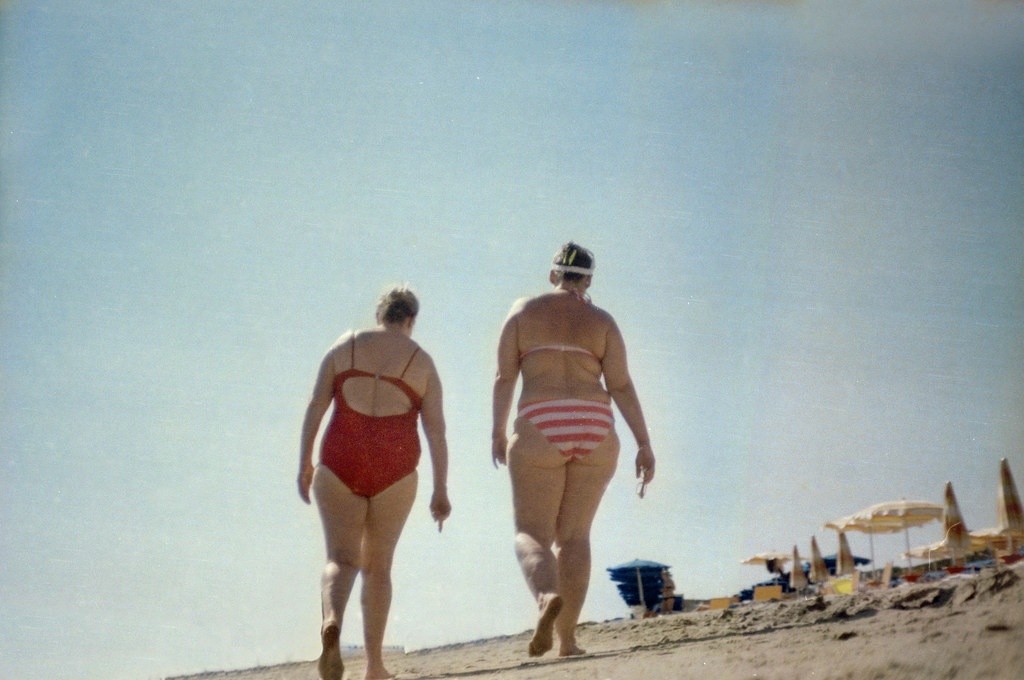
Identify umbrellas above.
[605,558,673,611]
[733,457,1024,602]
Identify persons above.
[297,283,452,680]
[492,242,654,657]
[661,572,675,615]
